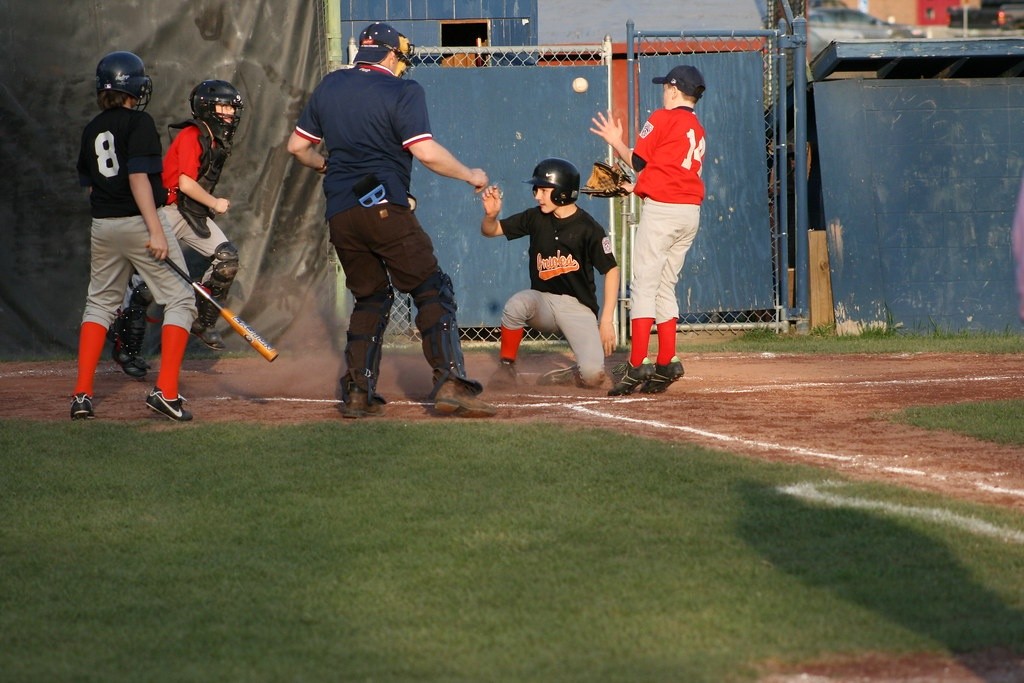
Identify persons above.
[70,51,198,422]
[106,79,244,378]
[287,22,497,419]
[589,64,707,397]
[481,157,620,392]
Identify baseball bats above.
[145,240,280,363]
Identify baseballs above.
[572,77,589,93]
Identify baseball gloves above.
[566,171,636,204]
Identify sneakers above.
[434,379,497,418]
[536,364,585,388]
[190,319,225,351]
[111,341,147,377]
[608,357,657,396]
[146,386,193,422]
[343,384,387,418]
[488,358,525,390]
[70,392,95,421]
[639,355,684,394]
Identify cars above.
[949,0,1023,30]
[808,8,927,39]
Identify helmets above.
[521,159,580,206]
[96,52,153,112]
[190,80,244,143]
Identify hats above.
[353,22,400,64]
[652,65,706,99]
